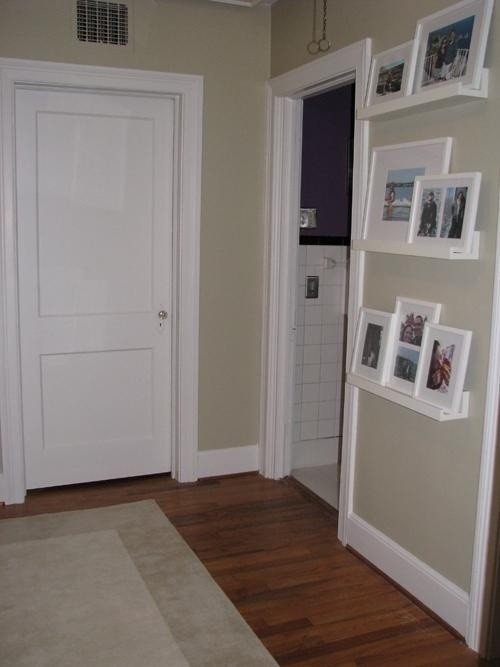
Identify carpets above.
[0,497,276,666]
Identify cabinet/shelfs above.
[345,64,489,422]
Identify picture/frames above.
[349,296,476,417]
[364,2,493,109]
[361,137,482,253]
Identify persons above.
[433,27,460,81]
[427,339,451,395]
[395,313,423,344]
[420,192,437,238]
[448,190,465,240]
[385,180,400,205]
